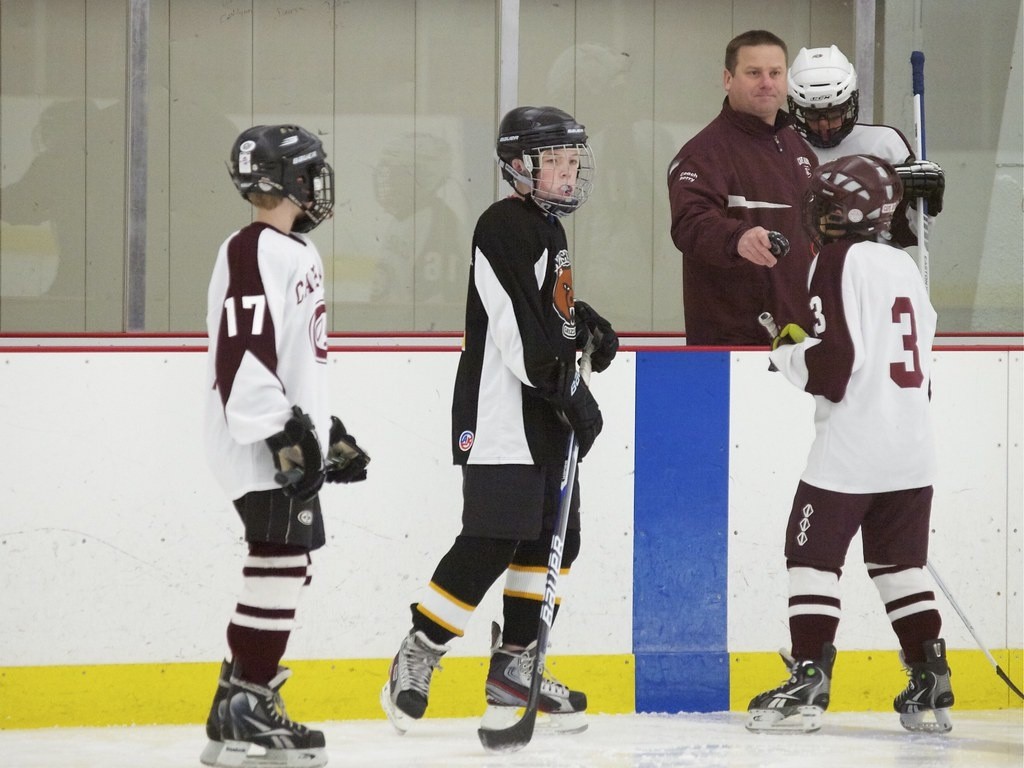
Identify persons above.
[788,42,946,251]
[380,107,618,733]
[200,123,372,768]
[667,31,820,346]
[744,155,956,736]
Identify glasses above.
[799,108,845,121]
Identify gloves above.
[321,414,370,485]
[265,406,326,502]
[769,320,811,371]
[574,300,619,373]
[549,361,603,460]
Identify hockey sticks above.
[910,50,932,403]
[758,310,1024,700]
[274,454,347,488]
[476,333,595,748]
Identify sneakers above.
[215,663,329,768]
[380,624,450,736]
[480,620,588,734]
[201,658,234,766]
[892,638,954,734]
[744,648,837,733]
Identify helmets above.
[231,123,335,232]
[800,154,903,250]
[785,45,860,149]
[496,106,596,217]
